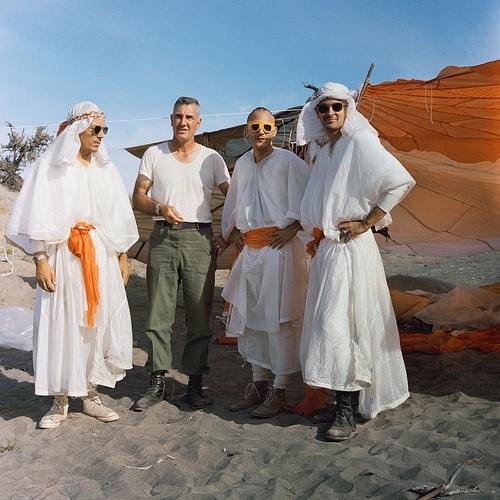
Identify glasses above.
[316,102,347,114]
[247,121,275,132]
[88,126,109,134]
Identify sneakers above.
[83,396,119,423]
[40,398,69,428]
[253,388,288,417]
[231,380,268,411]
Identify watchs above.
[155,204,161,216]
[33,255,46,263]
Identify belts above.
[154,220,211,229]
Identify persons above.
[131,97,231,411]
[297,82,416,440]
[5,102,140,429]
[220,107,311,418]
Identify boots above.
[135,370,167,410]
[310,410,335,425]
[328,392,362,438]
[187,375,210,410]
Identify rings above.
[342,230,346,235]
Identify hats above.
[35,101,109,162]
[297,82,378,147]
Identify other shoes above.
[218,336,237,344]
